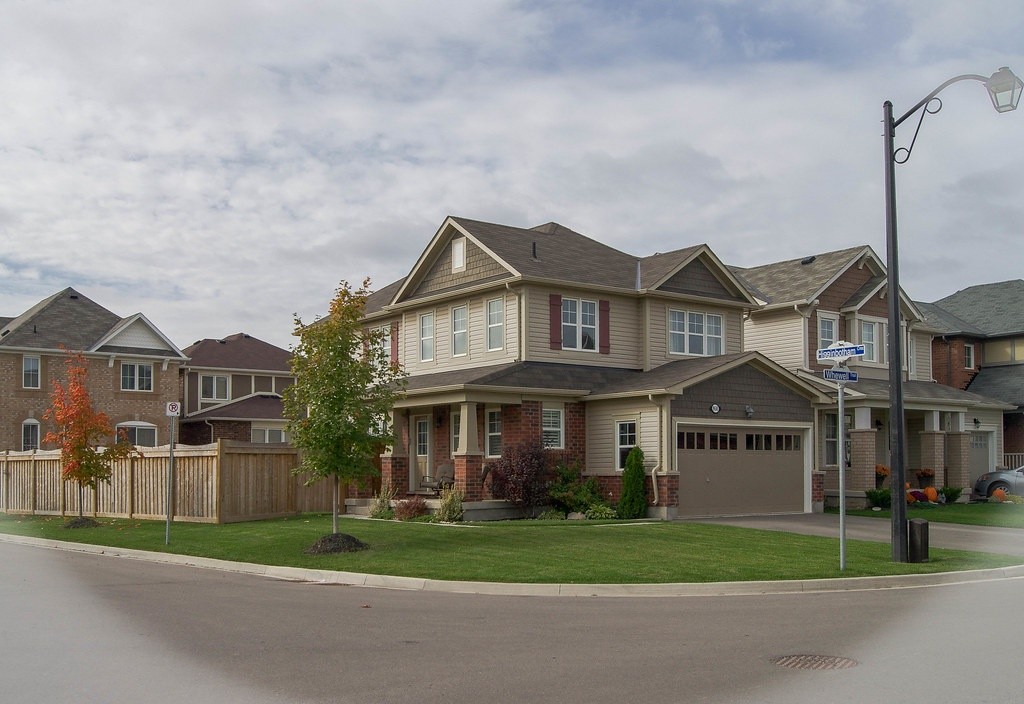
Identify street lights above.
[881,66,1024,562]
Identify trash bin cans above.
[909,518,929,563]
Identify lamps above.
[974,418,982,429]
[434,415,442,429]
[745,401,755,419]
[876,419,883,430]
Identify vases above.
[917,478,930,489]
[876,476,886,490]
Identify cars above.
[973,465,1024,502]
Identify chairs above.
[420,463,455,490]
[438,462,490,495]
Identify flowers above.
[875,464,890,477]
[916,468,935,481]
